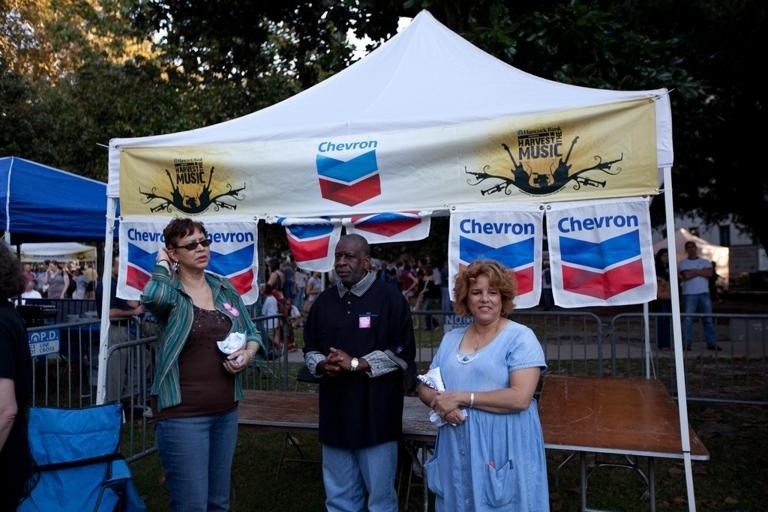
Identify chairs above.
[12,401,148,511]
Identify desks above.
[536,373,710,511]
[141,388,438,511]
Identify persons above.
[0,239,33,512]
[302,234,416,512]
[654,247,671,353]
[140,217,268,512]
[22,258,161,419]
[418,259,550,512]
[257,241,454,361]
[677,241,724,351]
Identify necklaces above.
[474,344,480,352]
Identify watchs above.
[350,357,359,372]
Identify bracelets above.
[471,392,475,408]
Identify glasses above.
[176,239,212,250]
